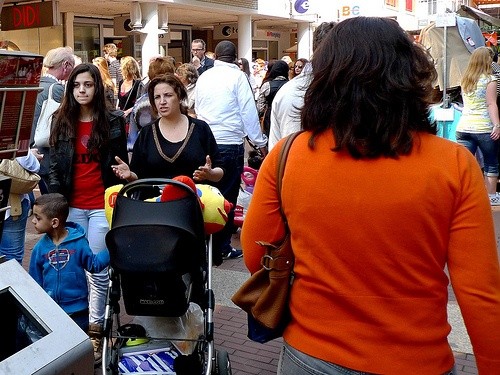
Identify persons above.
[177,39,307,269]
[454,46,500,206]
[239,16,500,375]
[268,22,337,153]
[110,73,224,201]
[29,194,109,335]
[1,149,40,267]
[28,44,176,195]
[50,63,127,367]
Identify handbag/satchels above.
[231,233,299,328]
[0,157,41,194]
[246,287,284,343]
[33,82,63,149]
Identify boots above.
[89,324,117,364]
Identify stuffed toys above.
[104,176,233,231]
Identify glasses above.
[191,48,205,51]
[239,62,243,65]
[295,64,303,69]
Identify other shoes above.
[227,246,245,259]
[488,191,500,206]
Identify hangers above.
[437,8,459,22]
[438,92,454,109]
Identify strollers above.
[234,137,266,231]
[92,177,233,375]
